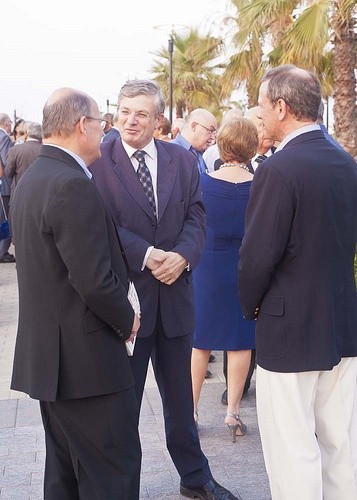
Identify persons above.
[153,99,343,405]
[87,78,237,500]
[100,113,120,142]
[0,112,42,262]
[8,87,143,500]
[238,64,357,500]
[189,117,259,443]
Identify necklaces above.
[220,163,249,171]
[160,135,168,140]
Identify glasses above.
[191,121,215,134]
[73,115,108,130]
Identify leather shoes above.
[180,477,239,500]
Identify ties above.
[133,149,157,223]
[255,155,268,167]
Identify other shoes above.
[0,253,16,263]
[221,387,248,405]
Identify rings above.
[165,278,167,282]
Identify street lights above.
[153,23,189,126]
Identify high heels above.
[193,411,199,435]
[224,413,247,443]
